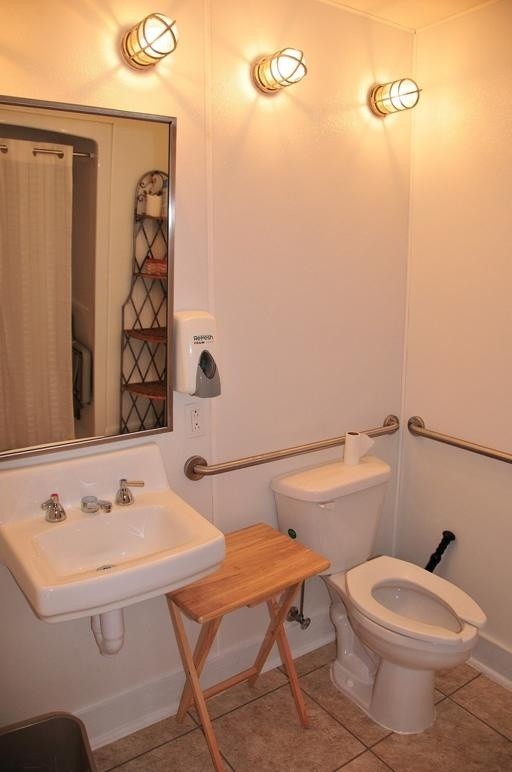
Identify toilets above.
[268,456,490,739]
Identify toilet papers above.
[340,429,377,465]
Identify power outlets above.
[182,404,211,438]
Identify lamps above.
[253,47,308,96]
[367,78,420,120]
[124,13,178,71]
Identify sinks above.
[0,440,228,625]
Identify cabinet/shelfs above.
[118,170,166,433]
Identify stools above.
[165,521,331,771]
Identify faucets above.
[79,494,113,515]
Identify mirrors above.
[2,94,178,461]
